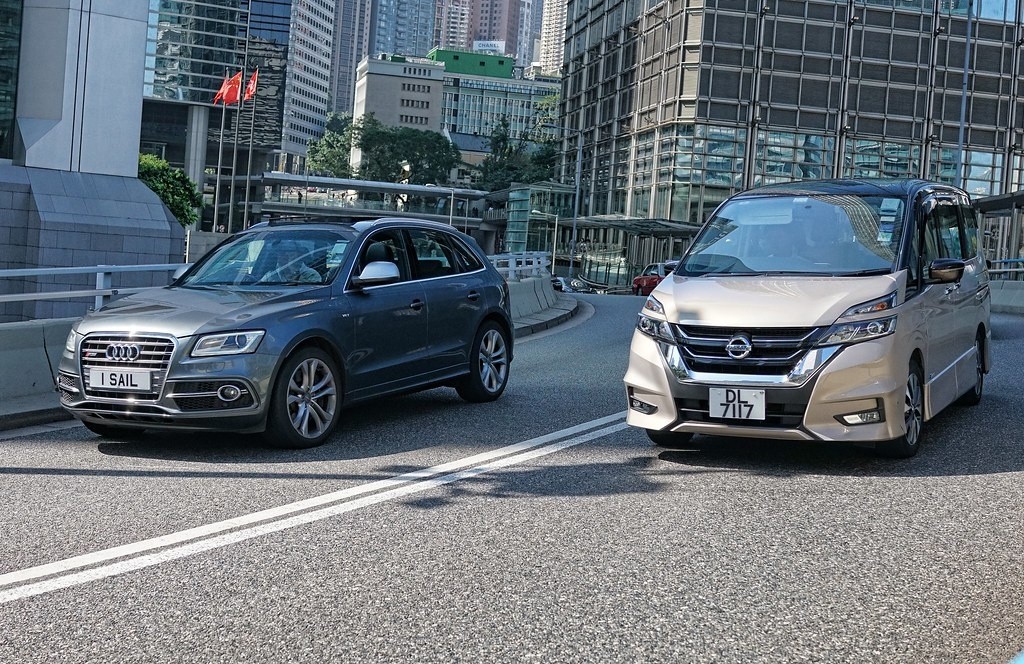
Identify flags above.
[244,70,257,103]
[214,71,242,106]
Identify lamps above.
[651,13,667,22]
[627,27,642,35]
[928,135,938,143]
[849,16,860,27]
[608,39,621,48]
[761,6,770,17]
[644,117,658,126]
[755,117,762,124]
[1009,143,1021,151]
[842,125,851,134]
[934,27,946,37]
[1018,37,1024,46]
[621,124,634,133]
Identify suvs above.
[620,177,993,461]
[55,218,515,450]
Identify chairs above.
[366,242,394,264]
[418,259,442,279]
[302,247,321,275]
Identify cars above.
[632,262,681,296]
[551,274,563,292]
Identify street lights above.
[532,210,558,274]
[447,195,469,234]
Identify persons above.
[259,239,323,283]
[298,191,494,217]
